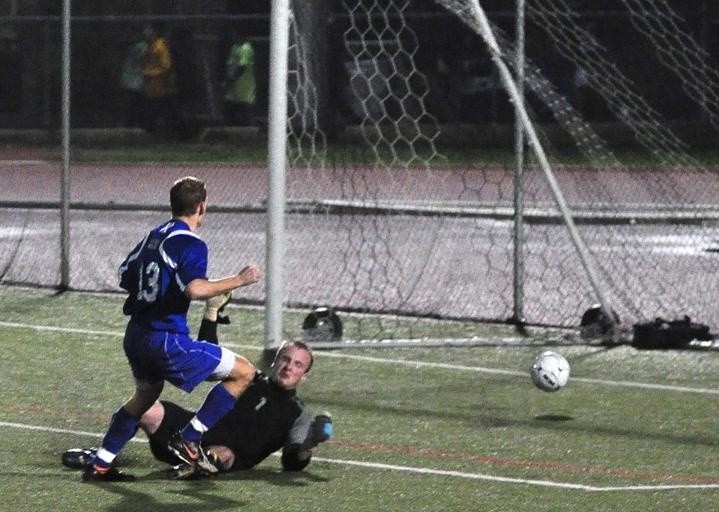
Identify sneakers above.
[62,447,135,483]
[166,430,219,481]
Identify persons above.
[218,18,267,151]
[60,284,336,476]
[136,22,191,145]
[82,173,261,488]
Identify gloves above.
[303,415,333,450]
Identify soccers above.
[530,351,570,392]
